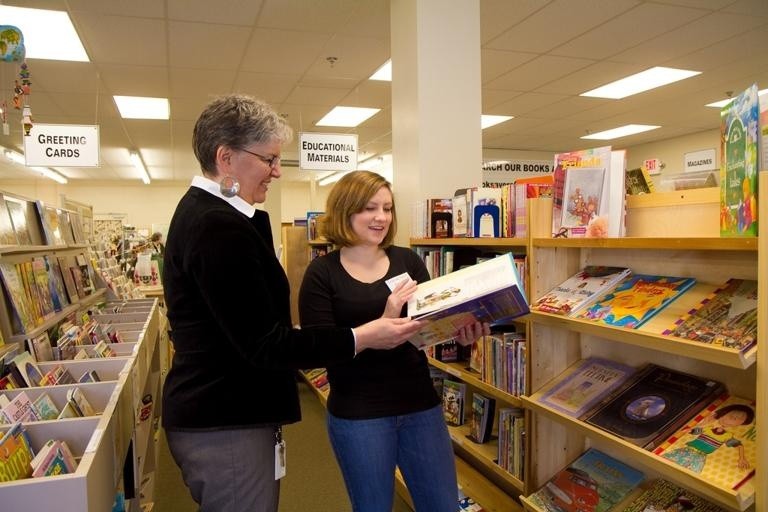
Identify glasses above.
[236,146,280,170]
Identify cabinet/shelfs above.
[518,172,765,511]
[0,191,167,512]
[406,183,534,503]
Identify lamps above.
[4,150,67,184]
[131,154,151,184]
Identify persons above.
[151,232,165,254]
[298,171,490,512]
[162,95,430,512]
